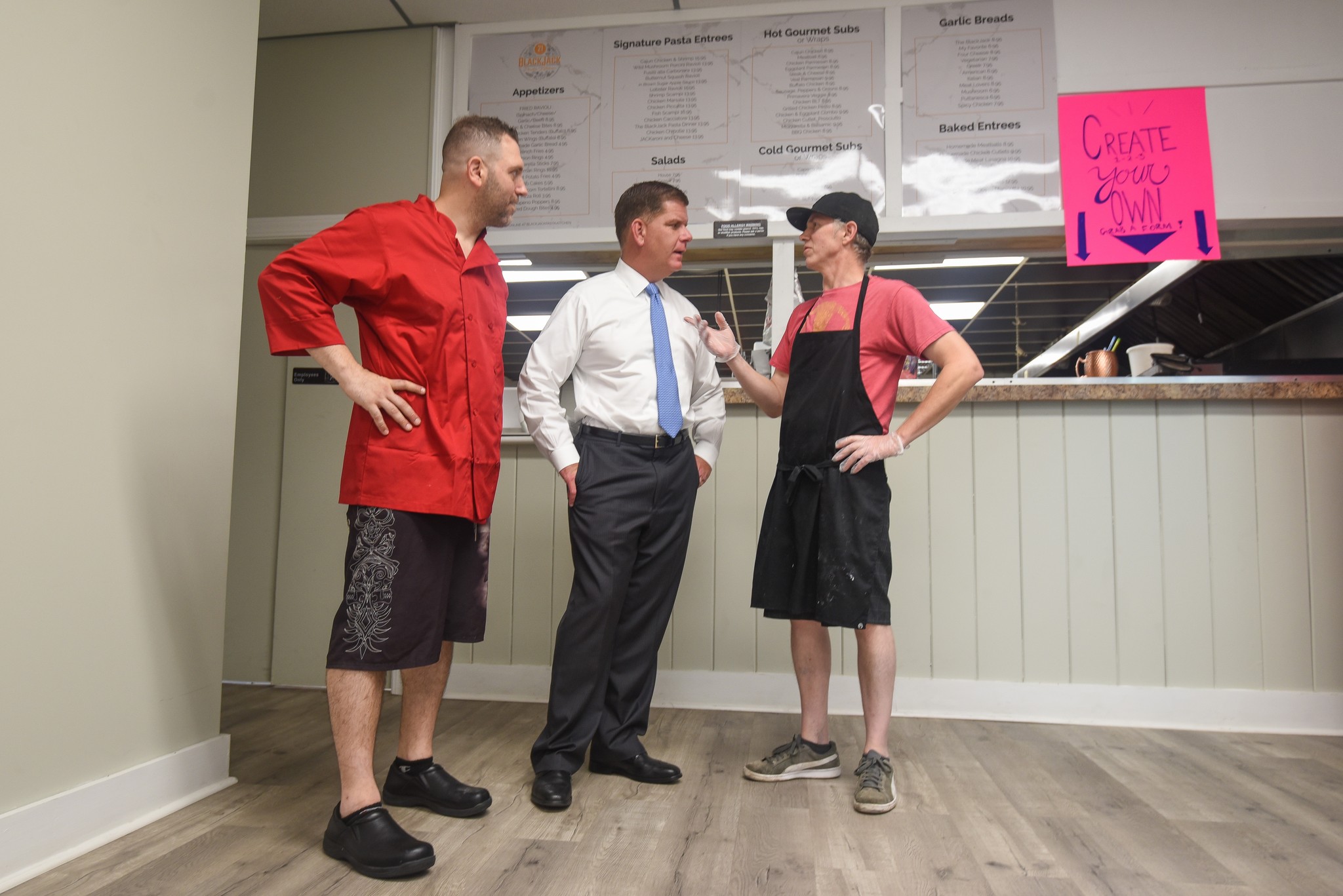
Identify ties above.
[644,281,683,440]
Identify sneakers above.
[743,734,842,782]
[853,749,898,814]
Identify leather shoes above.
[383,761,492,817]
[531,769,573,808]
[322,799,436,879]
[589,751,683,784]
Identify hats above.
[785,192,880,248]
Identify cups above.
[1126,342,1174,377]
[1075,350,1118,377]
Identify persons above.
[517,182,728,811]
[259,115,527,880]
[684,192,984,813]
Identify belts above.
[578,424,689,450]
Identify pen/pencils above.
[1103,346,1107,351]
[1111,337,1122,352]
[1107,336,1117,351]
